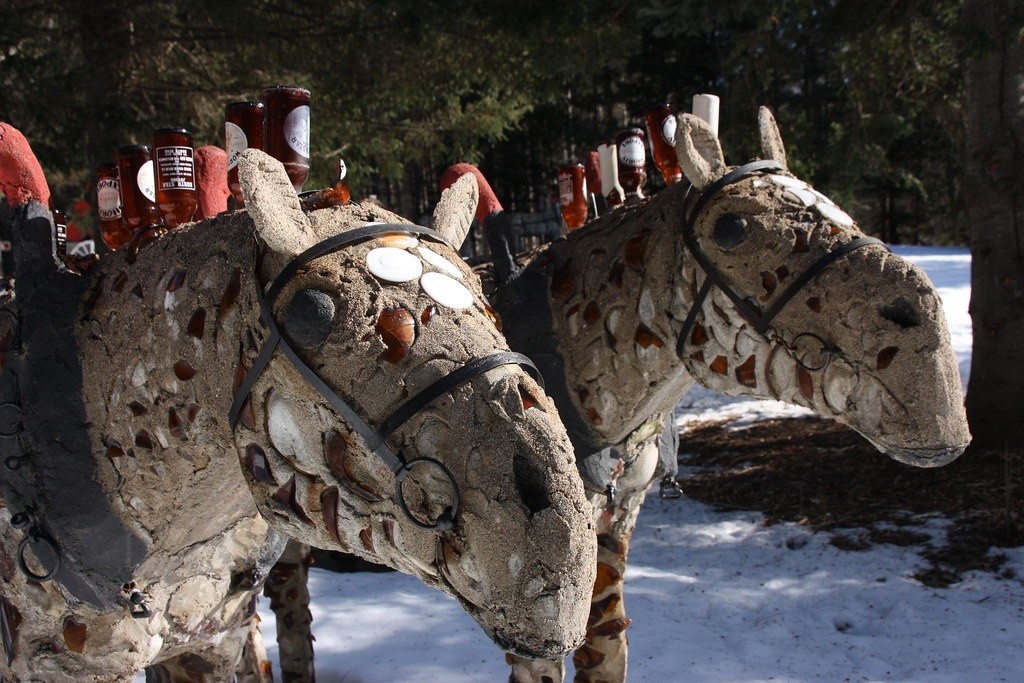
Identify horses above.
[1,103,972,678]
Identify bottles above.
[615,128,647,197]
[262,85,310,195]
[224,100,263,202]
[95,163,134,251]
[558,163,589,231]
[644,104,682,187]
[53,211,67,260]
[115,143,160,233]
[152,128,198,231]
